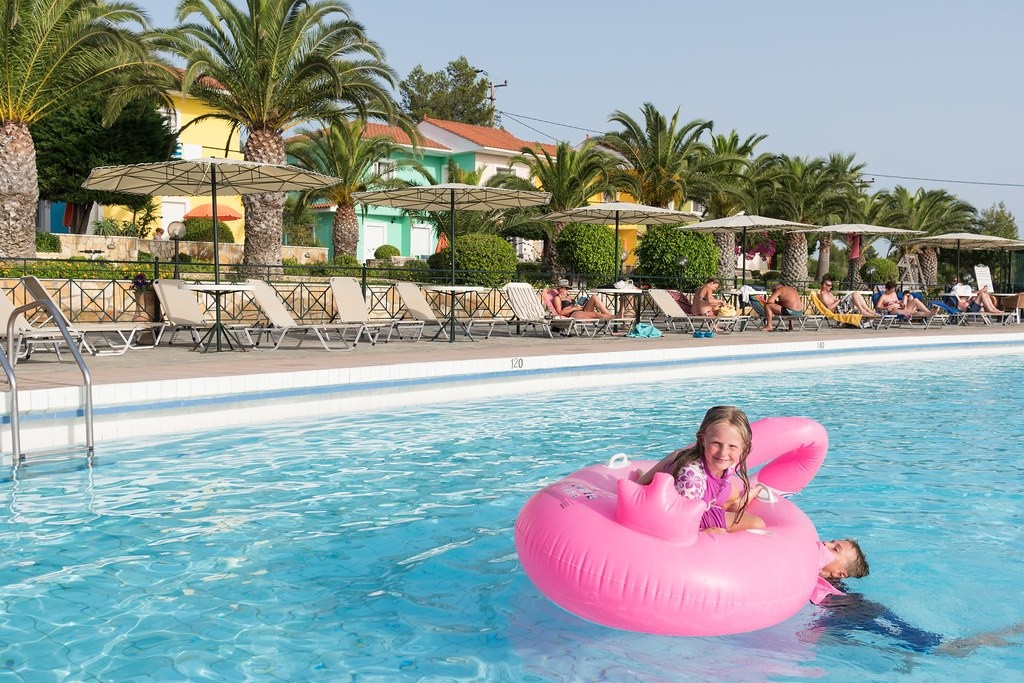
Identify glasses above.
[825,284,832,287]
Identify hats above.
[555,279,572,290]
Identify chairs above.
[0,276,1024,385]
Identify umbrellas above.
[80,155,345,352]
[529,200,702,334]
[787,224,929,290]
[350,180,552,339]
[672,215,824,335]
[182,202,243,221]
[893,231,1024,283]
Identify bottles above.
[884,307,888,315]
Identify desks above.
[187,283,253,351]
[589,288,642,335]
[423,285,485,343]
[833,290,874,295]
[992,293,1018,322]
[937,292,977,326]
[721,289,767,330]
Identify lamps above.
[620,250,628,261]
[865,265,876,275]
[168,222,187,239]
[674,256,690,269]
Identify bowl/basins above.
[613,284,626,288]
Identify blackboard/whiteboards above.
[974,263,995,294]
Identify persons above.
[818,280,881,317]
[692,278,725,332]
[876,282,940,316]
[153,226,164,240]
[760,283,803,332]
[552,280,625,319]
[820,538,869,580]
[958,285,1004,313]
[899,290,917,310]
[946,275,957,293]
[636,402,767,535]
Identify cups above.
[972,287,975,293]
[749,287,752,293]
[945,286,950,293]
[415,255,419,259]
[628,280,633,285]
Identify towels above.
[808,288,863,326]
[669,290,693,314]
[624,323,662,338]
[739,284,754,303]
[872,291,961,321]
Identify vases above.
[132,291,162,344]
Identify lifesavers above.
[514,416,847,638]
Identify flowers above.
[128,272,154,311]
